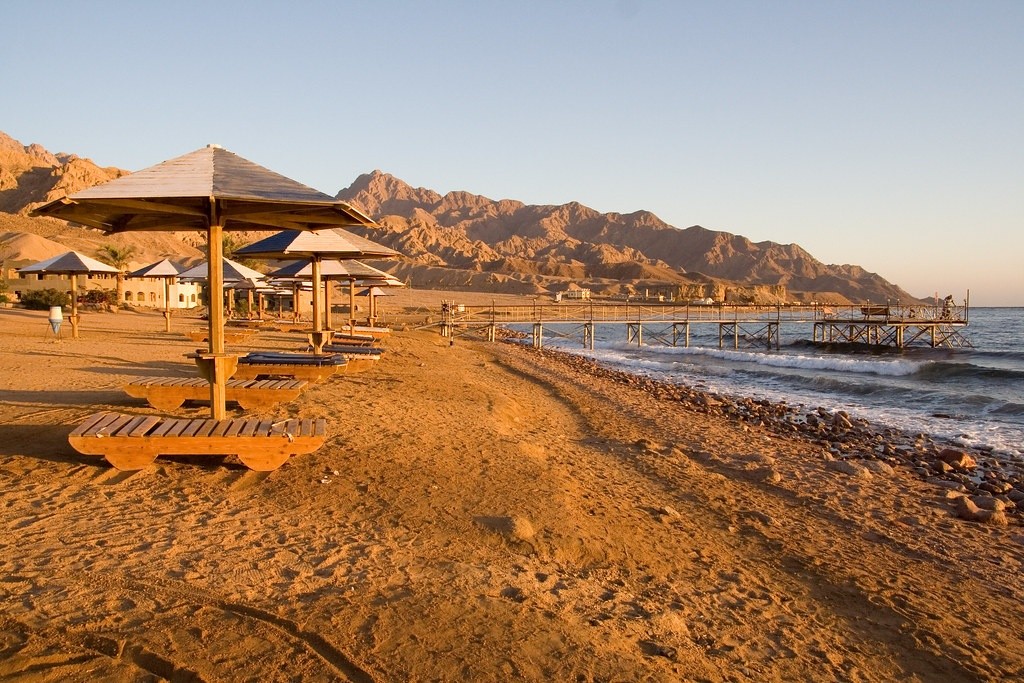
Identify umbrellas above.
[229,226,404,354]
[14,250,122,338]
[29,144,377,425]
[121,257,191,334]
[175,255,409,345]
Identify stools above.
[124,374,309,412]
[222,313,390,382]
[68,413,327,471]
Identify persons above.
[940,294,957,321]
